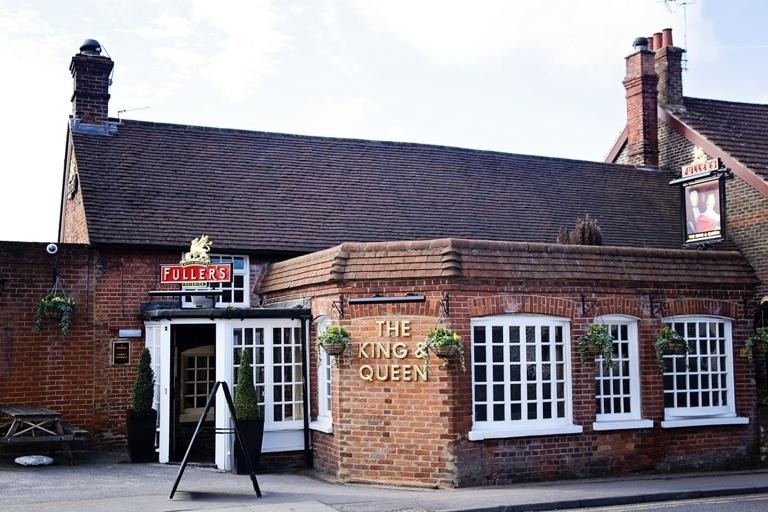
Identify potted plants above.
[314,322,353,369]
[422,327,467,373]
[125,346,157,463]
[33,293,76,336]
[575,323,616,368]
[233,350,264,474]
[652,324,689,375]
[744,309,768,363]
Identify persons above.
[687,188,704,234]
[697,191,719,232]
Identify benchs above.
[43,424,89,466]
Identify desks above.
[0,405,75,466]
